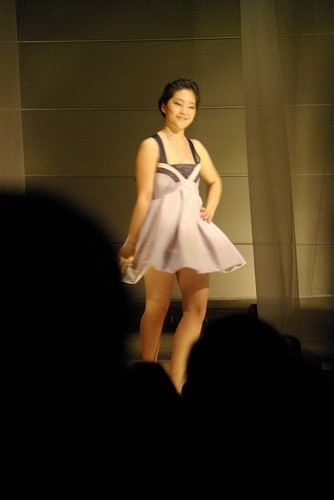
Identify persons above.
[3,180,334,497]
[114,78,248,396]
